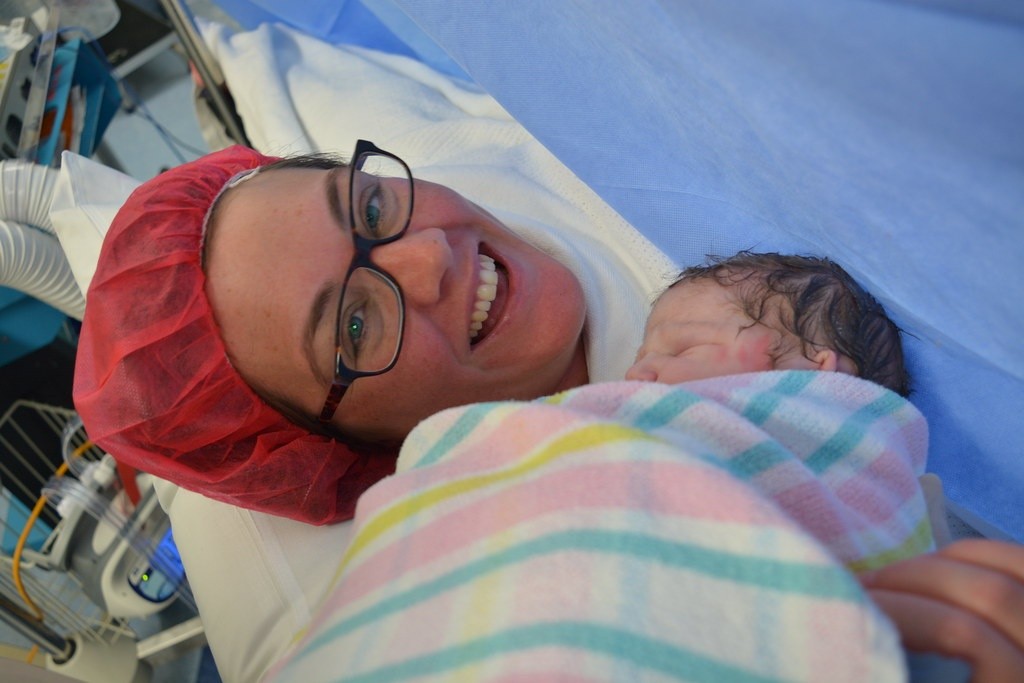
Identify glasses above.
[318,139,414,426]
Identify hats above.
[71,147,397,528]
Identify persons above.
[73,140,1024,683]
[269,249,938,683]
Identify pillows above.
[51,150,357,683]
